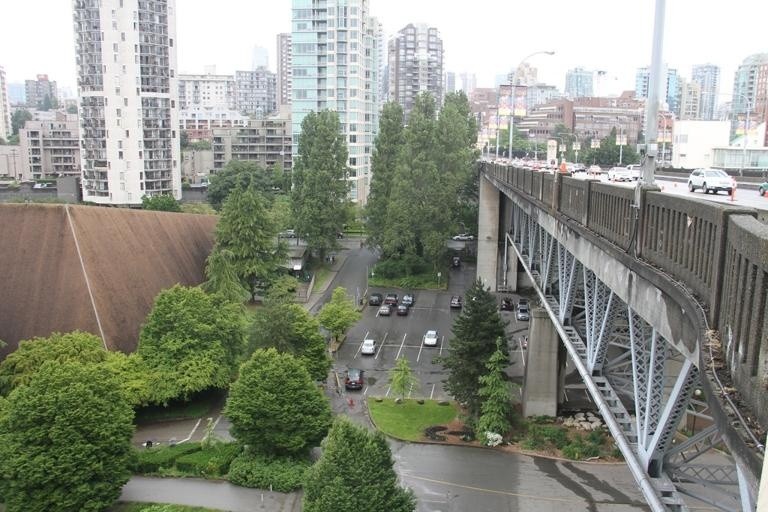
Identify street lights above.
[508,48,556,162]
[546,92,752,168]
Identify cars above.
[361,339,377,354]
[277,228,298,239]
[500,296,514,310]
[495,154,643,183]
[453,233,474,242]
[344,368,364,391]
[517,297,531,348]
[424,330,439,346]
[369,292,415,315]
[687,167,737,196]
[450,295,463,309]
